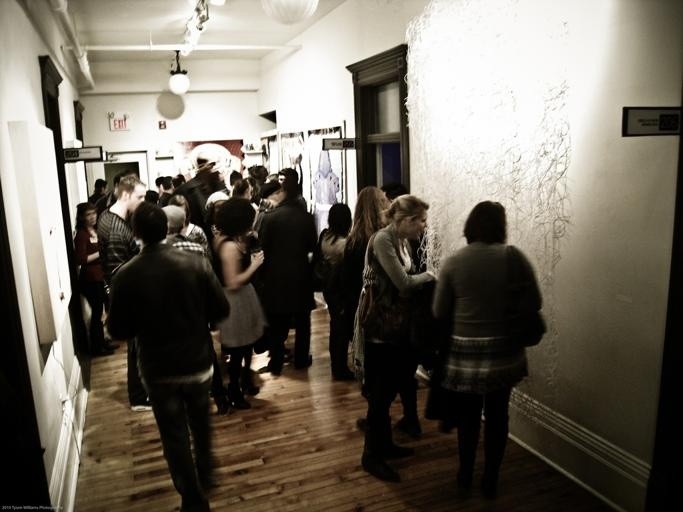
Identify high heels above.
[241,381,259,395]
[227,384,251,409]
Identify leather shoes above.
[361,458,399,480]
[357,418,367,427]
[384,445,414,461]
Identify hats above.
[161,205,186,228]
[205,191,229,211]
[248,165,268,181]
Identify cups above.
[250,247,261,257]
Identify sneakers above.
[131,394,153,411]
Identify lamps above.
[168,50,190,95]
[179,0,226,58]
[260,0,319,26]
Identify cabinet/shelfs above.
[41,308,88,512]
[8,119,72,344]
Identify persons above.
[89,179,107,203]
[267,167,299,206]
[253,177,319,374]
[249,165,281,198]
[205,171,230,239]
[212,197,266,411]
[104,200,232,512]
[97,176,153,413]
[205,191,230,240]
[245,177,274,210]
[352,193,437,483]
[317,203,358,382]
[173,154,230,238]
[98,173,127,211]
[346,186,423,434]
[252,178,281,233]
[168,194,209,252]
[431,200,543,501]
[161,205,231,419]
[155,177,164,196]
[73,201,120,356]
[231,179,260,239]
[159,176,175,206]
